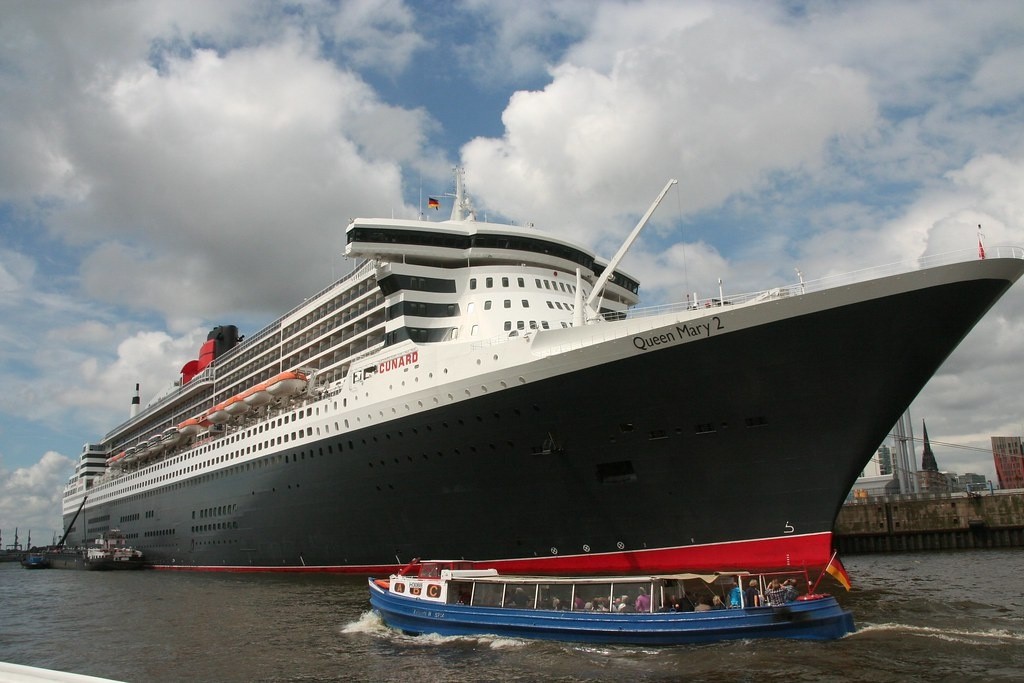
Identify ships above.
[63,163,1024,574]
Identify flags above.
[428,197,440,208]
[827,552,853,591]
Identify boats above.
[134,441,150,457]
[265,372,308,397]
[242,385,270,405]
[147,435,164,451]
[368,557,857,642]
[222,396,252,416]
[161,428,186,445]
[76,527,145,571]
[124,447,138,462]
[205,404,228,424]
[105,458,118,467]
[177,418,199,435]
[113,453,125,464]
[20,496,88,568]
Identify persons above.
[467,578,801,616]
